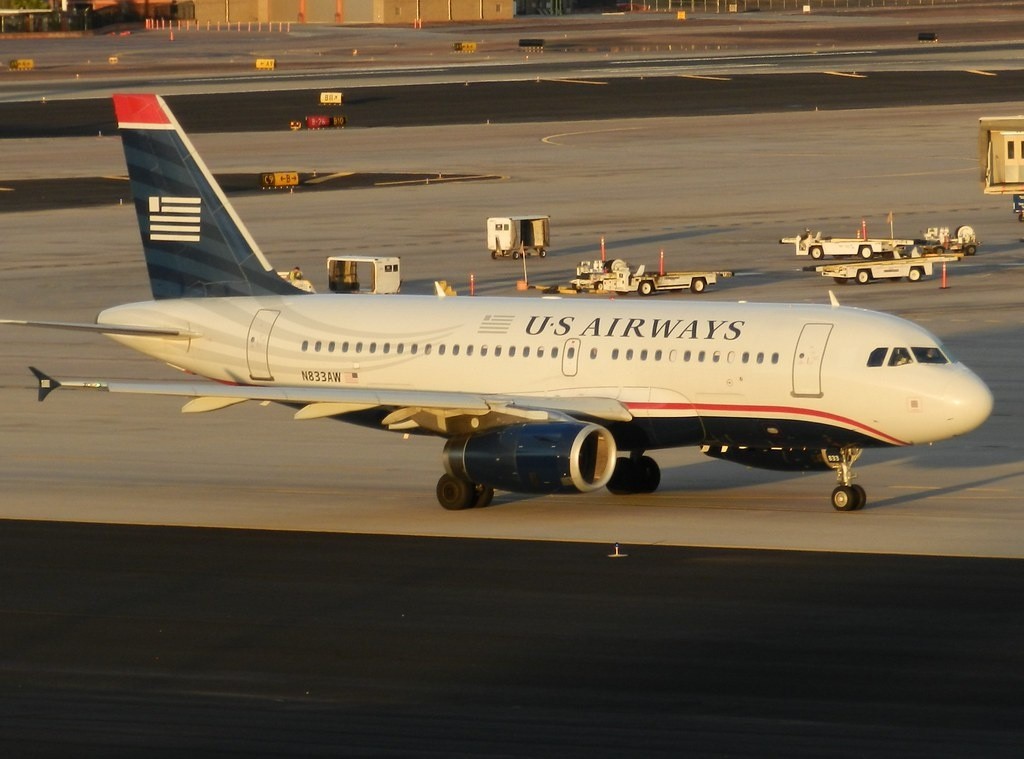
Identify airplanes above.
[0,91,996,510]
